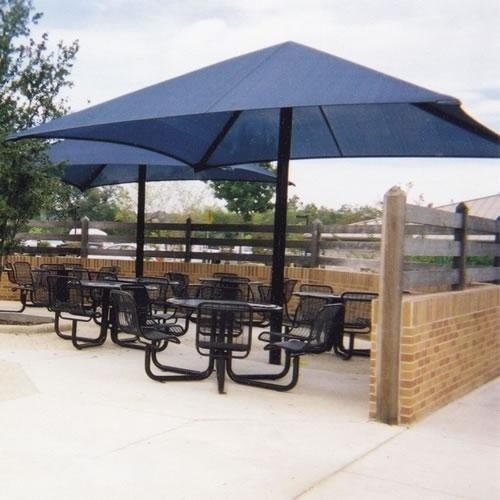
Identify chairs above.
[340,290,378,339]
[190,270,299,322]
[258,294,331,345]
[299,283,334,314]
[0,261,189,351]
[264,302,346,365]
[195,299,255,365]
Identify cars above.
[24,224,223,263]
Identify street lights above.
[296,214,316,258]
